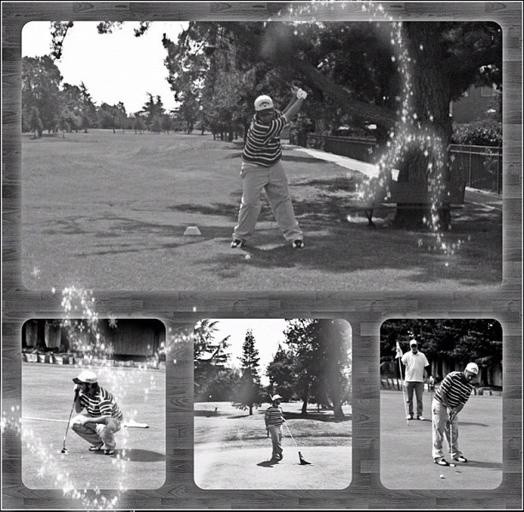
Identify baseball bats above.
[203,42,309,98]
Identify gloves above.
[295,88,308,100]
[430,376,435,385]
[291,86,299,95]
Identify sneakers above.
[292,240,304,248]
[455,456,467,462]
[417,416,426,420]
[104,449,115,455]
[89,442,104,451]
[231,239,245,249]
[434,458,449,466]
[407,414,413,420]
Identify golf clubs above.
[450,415,455,469]
[60,391,79,452]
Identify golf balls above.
[440,475,445,479]
[243,254,249,260]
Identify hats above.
[466,362,479,374]
[410,339,418,348]
[272,394,282,401]
[72,371,98,385]
[254,94,274,112]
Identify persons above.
[264,394,286,461]
[395,339,430,420]
[231,85,306,249]
[431,362,479,466]
[68,371,123,454]
